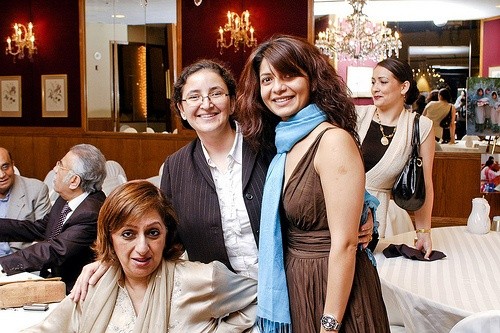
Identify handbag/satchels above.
[0,277,66,310]
[440,105,452,128]
[392,113,425,211]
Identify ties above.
[54,202,71,239]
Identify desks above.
[373,226,500,333]
[0,272,67,333]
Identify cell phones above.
[24,303,49,310]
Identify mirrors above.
[382,19,481,145]
[78,0,182,139]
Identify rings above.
[368,235,373,242]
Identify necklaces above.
[375,108,395,145]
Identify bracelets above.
[416,229,431,234]
[450,136,455,138]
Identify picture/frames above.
[41,74,68,118]
[0,76,22,118]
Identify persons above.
[0,148,53,257]
[351,59,434,260]
[233,34,391,333]
[41,182,259,333]
[481,156,500,192]
[0,144,107,296]
[471,88,500,133]
[157,59,374,282]
[412,88,467,144]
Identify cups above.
[491,216,500,231]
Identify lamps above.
[4,0,38,64]
[216,0,258,55]
[314,0,402,61]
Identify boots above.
[475,125,484,133]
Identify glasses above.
[57,161,69,172]
[181,92,231,106]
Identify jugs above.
[467,197,491,234]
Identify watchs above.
[319,315,342,331]
